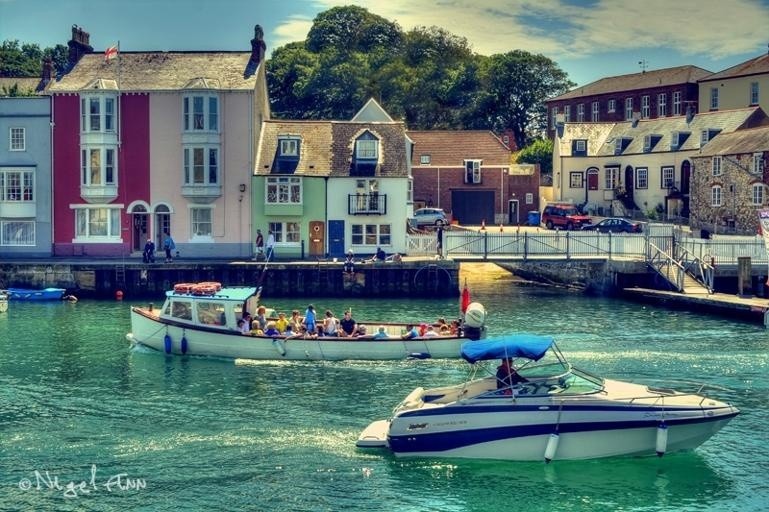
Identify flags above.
[105,42,119,62]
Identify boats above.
[359,336,742,465]
[8,285,68,303]
[129,281,507,363]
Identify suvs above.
[541,203,593,231]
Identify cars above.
[582,218,643,235]
[407,205,448,227]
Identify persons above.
[495,357,530,396]
[163,230,175,263]
[437,224,445,259]
[238,303,460,343]
[386,253,402,261]
[265,230,275,260]
[361,247,386,263]
[142,238,155,263]
[342,248,356,276]
[250,229,267,262]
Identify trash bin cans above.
[528,211,541,225]
[727,219,736,234]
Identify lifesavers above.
[173,282,223,296]
